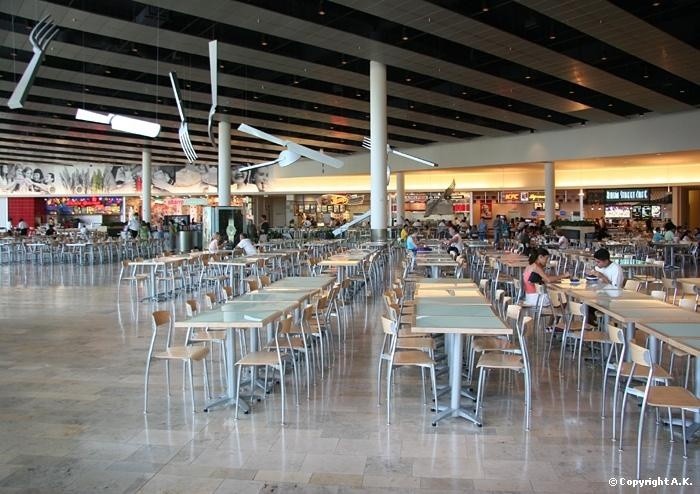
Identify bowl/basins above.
[604,289,623,297]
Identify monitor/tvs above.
[604,204,632,218]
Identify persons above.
[140,220,151,240]
[19,219,28,236]
[302,215,317,228]
[181,220,186,226]
[412,219,422,226]
[400,224,408,241]
[407,230,432,278]
[464,216,466,219]
[597,228,611,241]
[455,217,460,225]
[128,212,140,239]
[209,232,224,253]
[502,217,527,237]
[553,230,568,249]
[440,225,464,255]
[492,215,502,251]
[584,248,624,326]
[528,219,536,227]
[0,163,54,194]
[522,248,570,332]
[455,224,476,240]
[448,220,453,225]
[392,217,410,227]
[522,229,534,256]
[254,167,269,191]
[158,217,166,252]
[480,204,491,218]
[230,164,259,194]
[168,219,176,252]
[260,214,269,242]
[665,219,676,230]
[328,217,346,228]
[646,216,654,233]
[423,225,430,235]
[652,227,663,241]
[439,219,446,227]
[81,225,89,235]
[664,228,674,241]
[6,218,13,236]
[235,233,257,255]
[478,217,488,241]
[541,220,544,226]
[100,164,143,194]
[45,226,57,239]
[625,219,631,228]
[674,226,699,241]
[461,219,467,227]
[151,176,218,195]
[595,216,604,228]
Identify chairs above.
[558,295,618,392]
[619,338,700,481]
[476,316,534,434]
[236,316,299,425]
[544,233,700,278]
[183,300,227,400]
[611,289,667,349]
[201,294,245,372]
[387,304,437,404]
[262,304,317,406]
[479,279,489,297]
[666,344,699,390]
[601,323,675,444]
[0,228,163,265]
[466,305,534,408]
[460,289,506,371]
[282,296,330,380]
[143,310,210,413]
[545,287,597,368]
[466,297,520,381]
[644,299,699,362]
[380,290,431,352]
[376,313,442,426]
[215,285,253,361]
[593,278,642,330]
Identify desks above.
[412,238,459,279]
[174,275,337,414]
[412,288,490,305]
[0,233,160,265]
[480,247,544,301]
[122,247,235,305]
[317,235,395,332]
[315,247,377,306]
[414,277,478,289]
[410,304,513,429]
[208,238,316,287]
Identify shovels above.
[75,107,160,138]
[238,122,344,169]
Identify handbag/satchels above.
[448,245,460,256]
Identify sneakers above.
[544,326,562,334]
